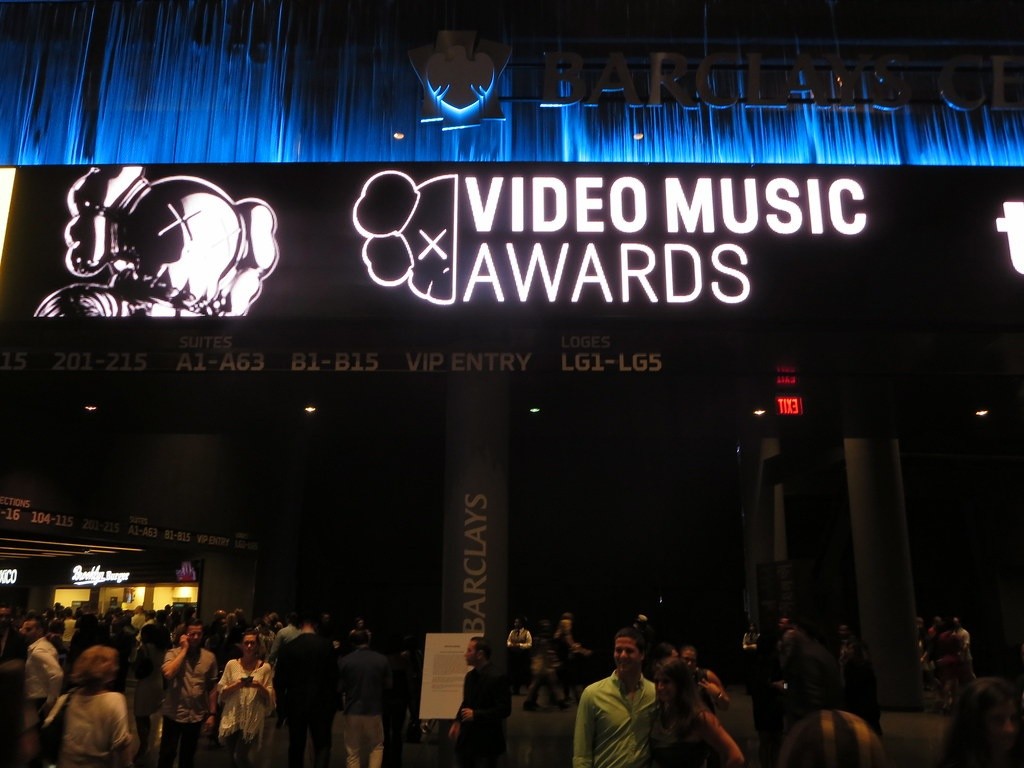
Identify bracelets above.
[717,693,724,701]
[209,712,216,716]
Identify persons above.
[448,637,513,768]
[0,595,1024,768]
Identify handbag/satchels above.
[137,669,165,704]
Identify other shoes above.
[523,700,539,710]
[132,747,150,763]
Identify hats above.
[637,614,648,622]
